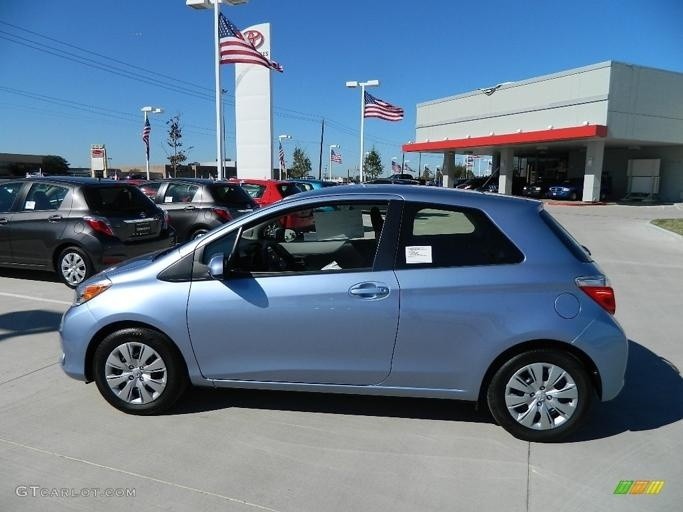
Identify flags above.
[362,91,405,122]
[279,142,286,169]
[330,149,342,164]
[217,11,284,73]
[391,160,401,173]
[141,116,152,160]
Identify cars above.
[59,183,630,443]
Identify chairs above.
[318,209,384,271]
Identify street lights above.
[329,144,440,184]
[279,135,292,181]
[346,79,380,184]
[141,106,165,180]
[185,0,248,180]
